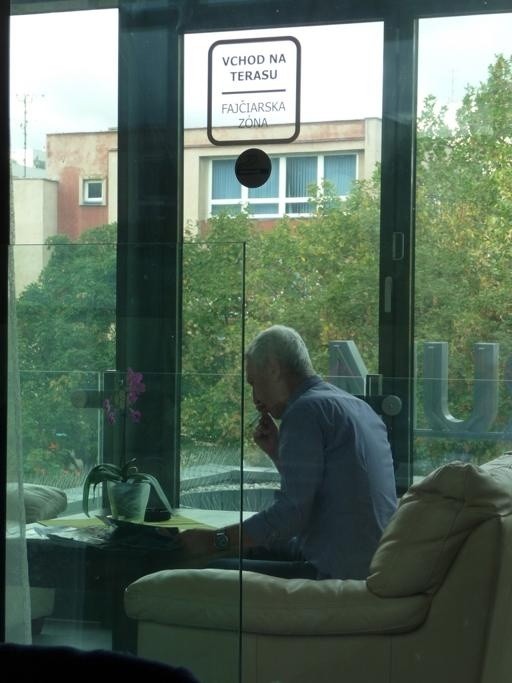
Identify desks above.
[5,504,260,654]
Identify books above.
[47,524,115,550]
[8,520,52,541]
[98,514,188,550]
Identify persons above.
[84,322,399,655]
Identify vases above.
[106,479,152,525]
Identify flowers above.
[82,367,177,519]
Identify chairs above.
[124,451,512,682]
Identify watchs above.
[213,526,231,550]
